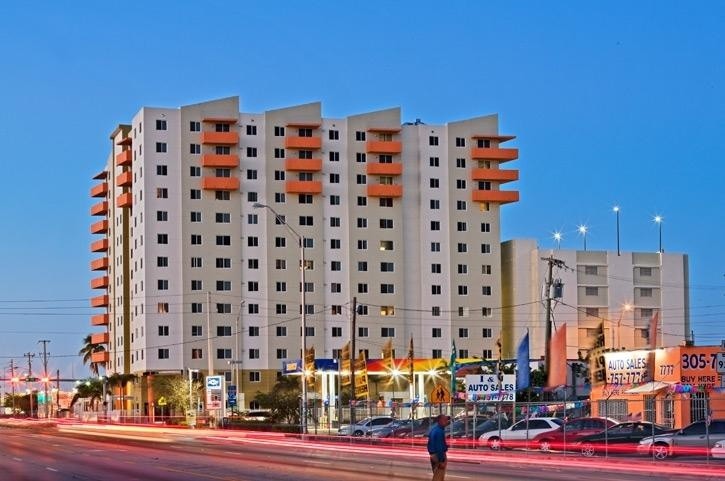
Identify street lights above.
[332,357,342,431]
[12,366,33,418]
[617,304,631,350]
[250,202,309,434]
[187,367,199,415]
[232,299,245,419]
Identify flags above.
[642,309,658,385]
[590,320,607,397]
[516,333,530,395]
[547,322,567,393]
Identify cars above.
[479,418,567,451]
[445,417,488,440]
[370,416,437,439]
[338,416,398,438]
[575,421,683,458]
[636,418,724,462]
[462,417,525,440]
[533,417,624,454]
[711,440,725,459]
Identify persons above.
[425,413,449,481]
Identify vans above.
[241,410,271,422]
